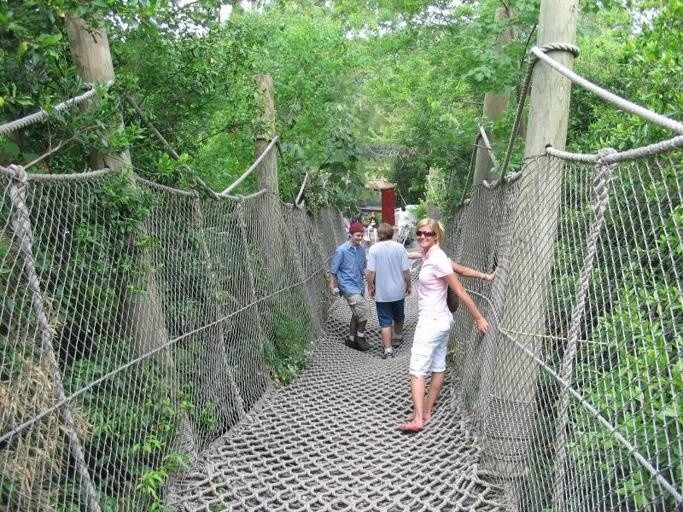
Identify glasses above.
[416,230,435,236]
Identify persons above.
[365,223,411,358]
[329,222,372,352]
[398,218,498,432]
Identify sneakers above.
[391,338,402,345]
[345,335,370,351]
[384,353,393,359]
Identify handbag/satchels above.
[447,287,459,313]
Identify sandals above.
[400,415,431,432]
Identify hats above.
[349,223,364,233]
